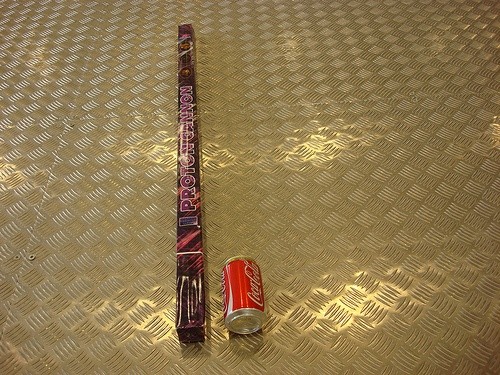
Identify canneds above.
[221,256,265,334]
